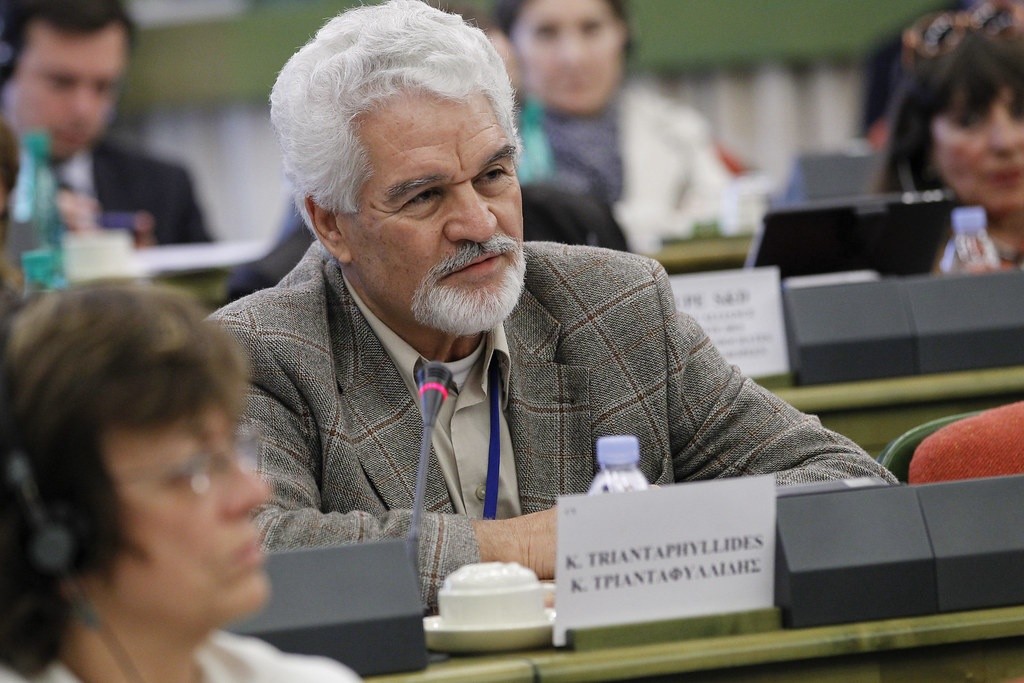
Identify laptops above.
[744,191,956,289]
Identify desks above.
[148,235,1024,683]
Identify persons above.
[199,0,901,612]
[0,277,362,683]
[0,0,228,284]
[863,0,1024,279]
[481,0,737,252]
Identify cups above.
[438,562,556,629]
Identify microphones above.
[404,360,453,555]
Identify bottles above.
[940,205,999,276]
[5,129,67,309]
[587,435,648,499]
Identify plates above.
[422,608,555,652]
